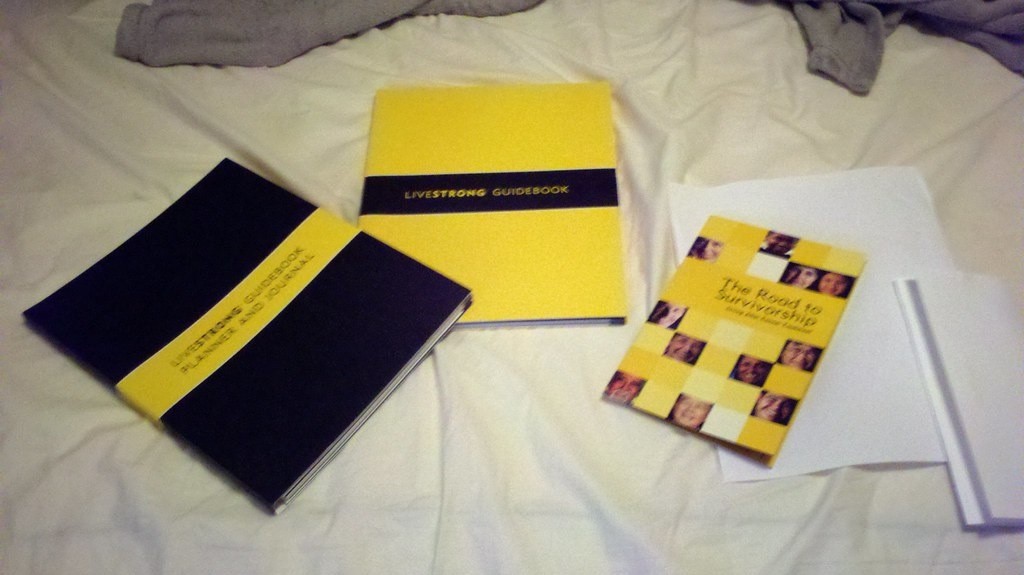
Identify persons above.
[647,301,688,329]
[751,390,797,425]
[816,271,855,299]
[729,355,773,387]
[689,237,724,263]
[663,333,706,366]
[777,340,822,372]
[666,393,713,433]
[778,262,825,291]
[605,371,645,406]
[759,231,798,259]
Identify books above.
[22,156,471,512]
[358,82,628,326]
[603,216,865,470]
